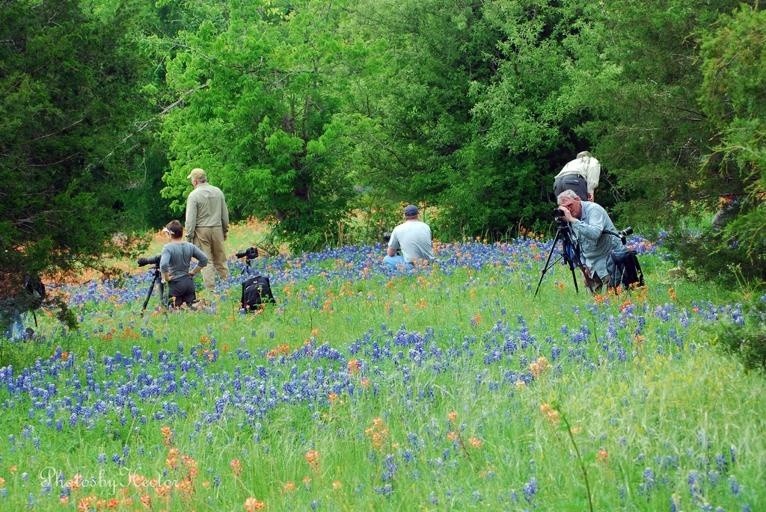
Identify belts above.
[407,262,429,266]
[567,173,584,180]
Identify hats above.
[162,225,176,235]
[187,167,208,182]
[405,205,420,216]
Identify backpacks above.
[605,250,646,296]
[240,275,277,314]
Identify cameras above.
[235,247,258,260]
[553,208,565,217]
[617,226,634,245]
[137,254,160,266]
[381,231,392,241]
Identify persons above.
[556,190,628,294]
[182,167,230,293]
[381,204,433,278]
[159,218,209,309]
[553,149,602,266]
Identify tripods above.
[533,234,595,295]
[140,271,164,317]
